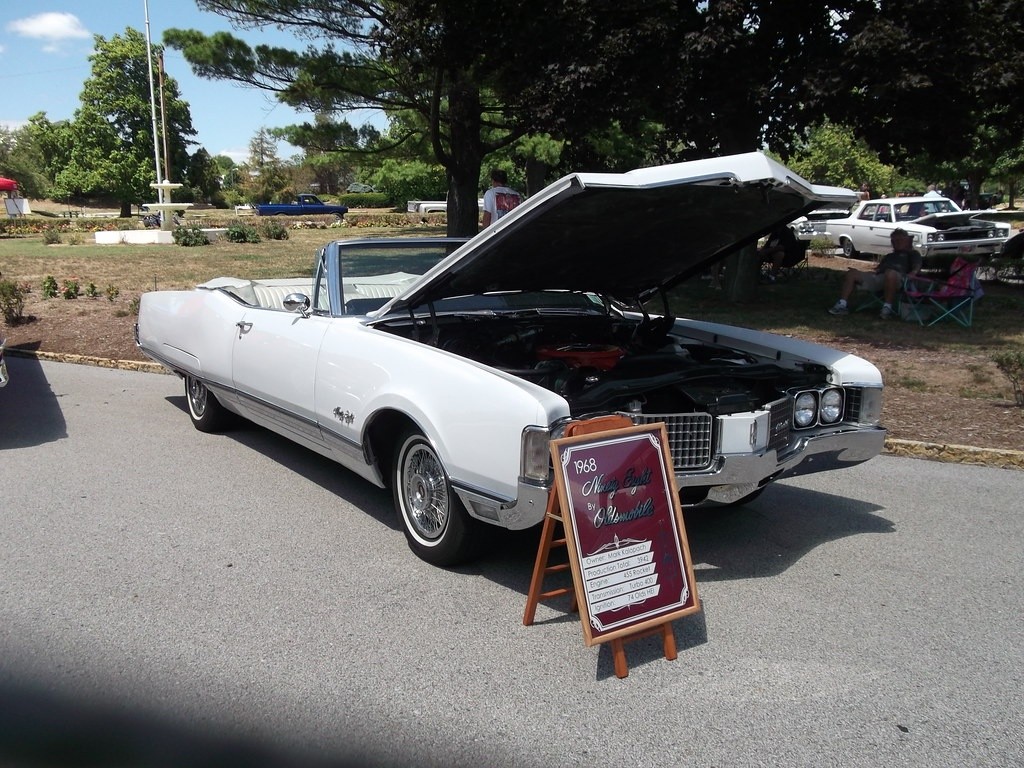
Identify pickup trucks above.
[251,193,349,223]
[405,197,487,216]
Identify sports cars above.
[134,148,890,571]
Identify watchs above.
[911,269,918,274]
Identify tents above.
[0,177,18,198]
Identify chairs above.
[897,258,979,326]
[853,234,927,315]
[253,282,328,315]
[353,283,404,298]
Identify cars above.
[824,194,1012,263]
[786,184,856,245]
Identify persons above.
[707,224,803,289]
[827,225,923,320]
[482,168,521,230]
[859,178,1003,218]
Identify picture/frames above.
[551,421,701,647]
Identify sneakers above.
[829,301,849,315]
[880,305,892,319]
